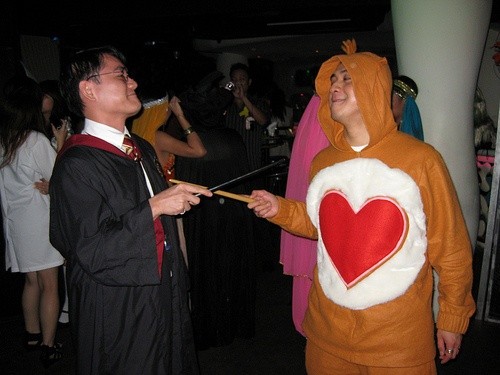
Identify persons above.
[0,62,424,369]
[239,38,478,375]
[48,46,214,375]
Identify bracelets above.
[184,126,194,135]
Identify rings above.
[181,210,186,214]
[447,349,453,354]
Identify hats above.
[392,75,419,102]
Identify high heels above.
[27,333,63,369]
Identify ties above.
[121,134,140,162]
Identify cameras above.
[225,81,234,91]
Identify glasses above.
[85,69,135,82]
